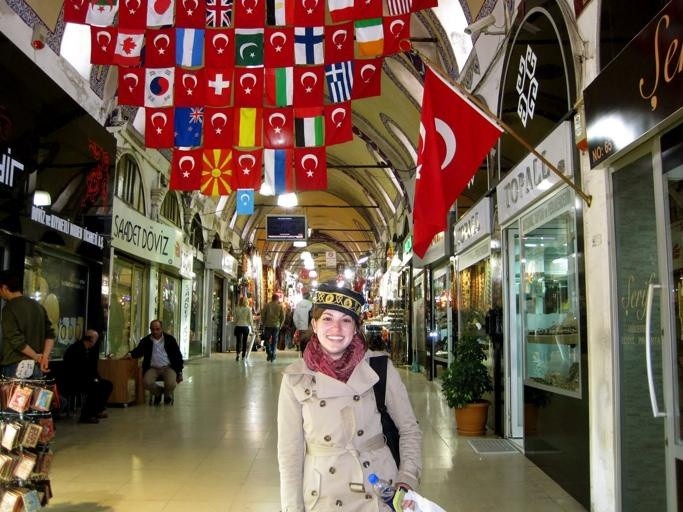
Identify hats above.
[313,284,364,319]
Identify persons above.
[62,329,113,424]
[124,320,184,406]
[277,283,422,512]
[0,269,55,411]
[232,291,314,363]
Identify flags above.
[265,149,328,195]
[118,58,382,109]
[411,62,505,259]
[236,189,254,216]
[145,101,355,149]
[63,1,438,68]
[169,148,262,196]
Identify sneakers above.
[236,356,240,361]
[154,387,163,406]
[165,398,172,404]
[90,418,99,424]
[266,351,276,363]
[99,414,108,418]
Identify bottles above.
[367,473,397,512]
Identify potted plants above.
[437,306,495,435]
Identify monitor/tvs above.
[264,213,308,242]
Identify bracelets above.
[36,353,42,362]
